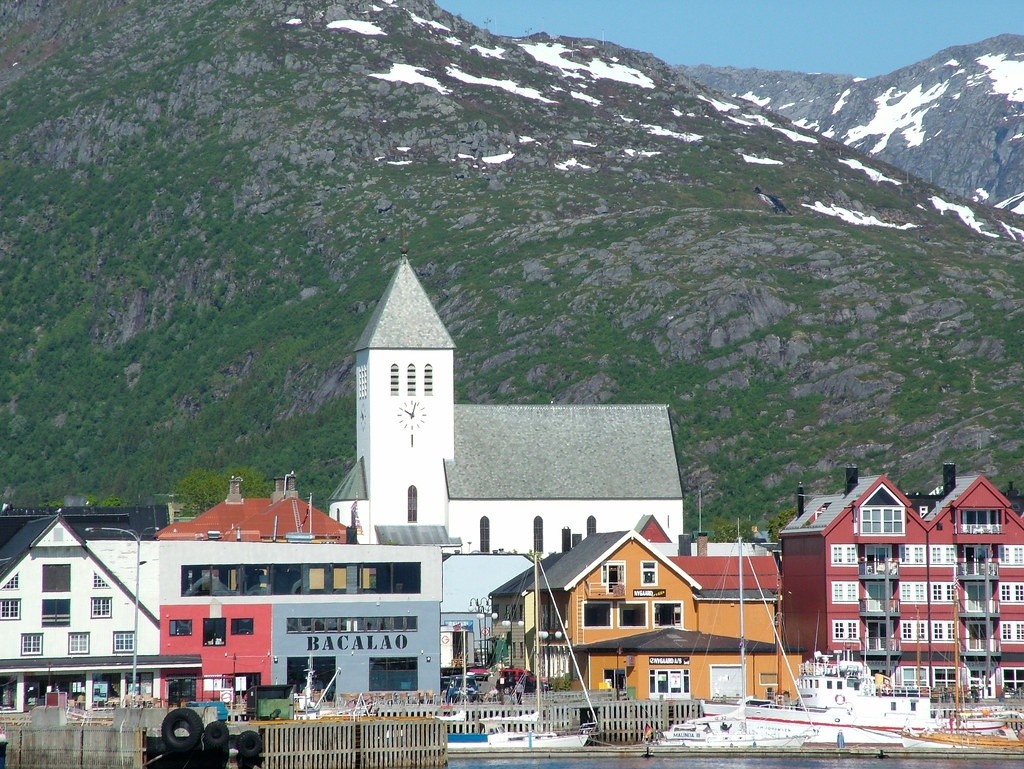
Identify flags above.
[481,628,490,636]
[453,623,461,631]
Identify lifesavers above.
[161,708,204,749]
[237,730,262,757]
[882,684,893,695]
[204,721,230,748]
[835,694,845,704]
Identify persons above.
[514,681,524,705]
[76,692,142,708]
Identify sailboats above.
[444,555,600,752]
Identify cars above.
[465,664,489,679]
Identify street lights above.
[468,598,492,667]
[501,618,524,665]
[476,605,498,665]
[83,525,160,705]
[538,631,562,692]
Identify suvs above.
[448,674,482,703]
[499,667,548,695]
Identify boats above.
[652,533,1008,748]
